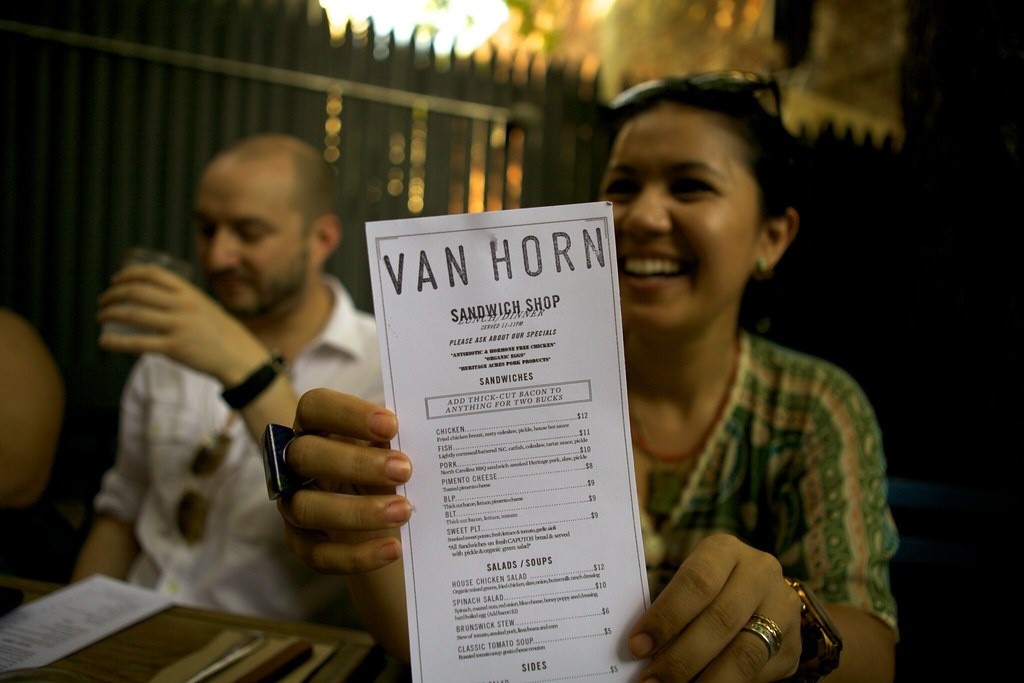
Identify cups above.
[101,246,205,336]
[0,668,92,683]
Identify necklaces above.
[641,329,750,566]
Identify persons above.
[281,75,900,683]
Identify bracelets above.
[220,352,284,410]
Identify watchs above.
[784,577,844,683]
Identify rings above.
[72,134,383,627]
[742,613,784,662]
[260,424,315,501]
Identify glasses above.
[176,412,237,546]
[607,67,782,118]
